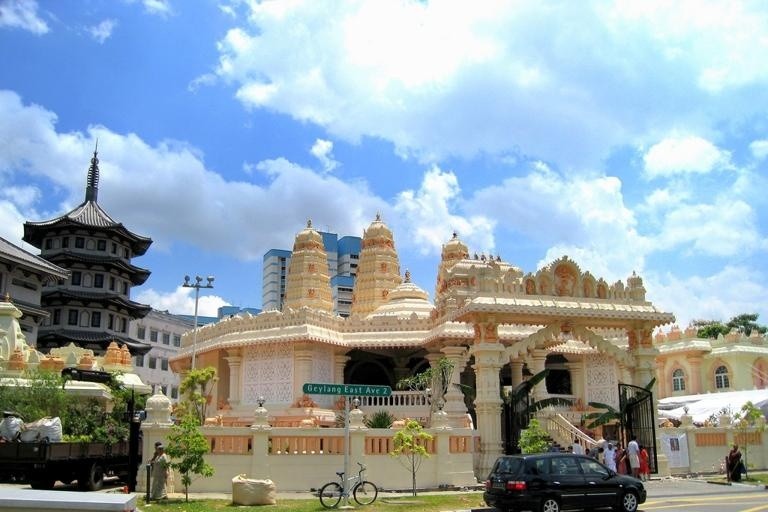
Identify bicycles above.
[320,462,378,509]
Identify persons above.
[728,445,741,482]
[564,434,650,481]
[148,442,162,463]
[151,445,172,500]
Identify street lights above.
[182,275,214,369]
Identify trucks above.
[0,410,176,491]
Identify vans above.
[483,452,646,512]
